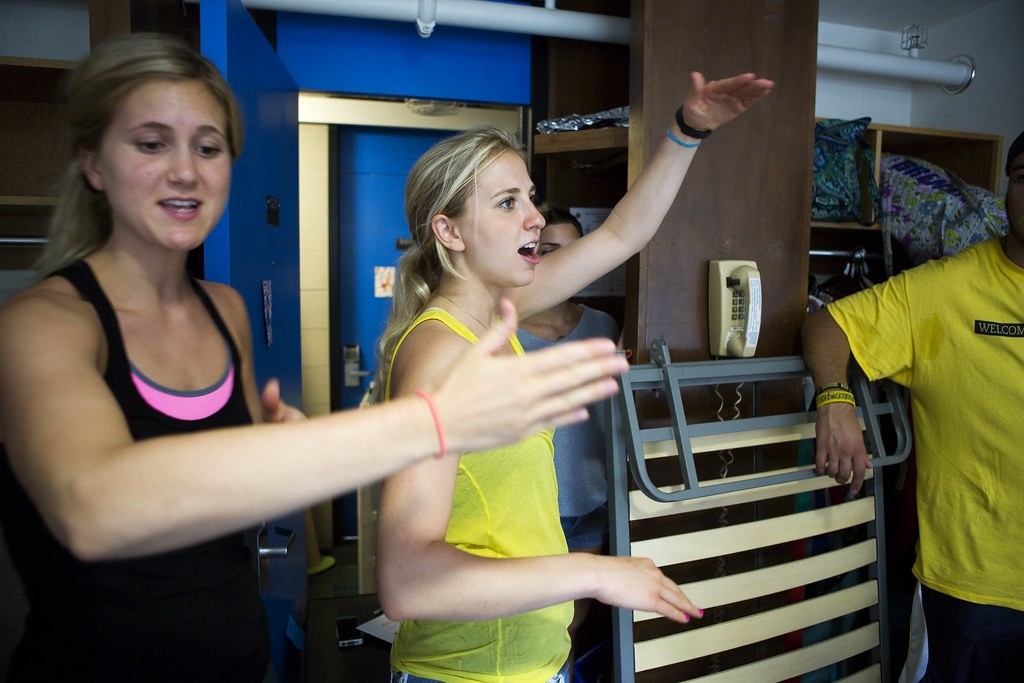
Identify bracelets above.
[417,392,445,459]
[675,106,712,139]
[815,383,856,408]
[666,129,700,148]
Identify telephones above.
[708,259,764,359]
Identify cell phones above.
[336,616,363,646]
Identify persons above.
[801,132,1024,683]
[512,211,630,675]
[377,71,774,683]
[0,33,628,683]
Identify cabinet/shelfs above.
[808,117,1004,311]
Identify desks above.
[306,593,390,683]
